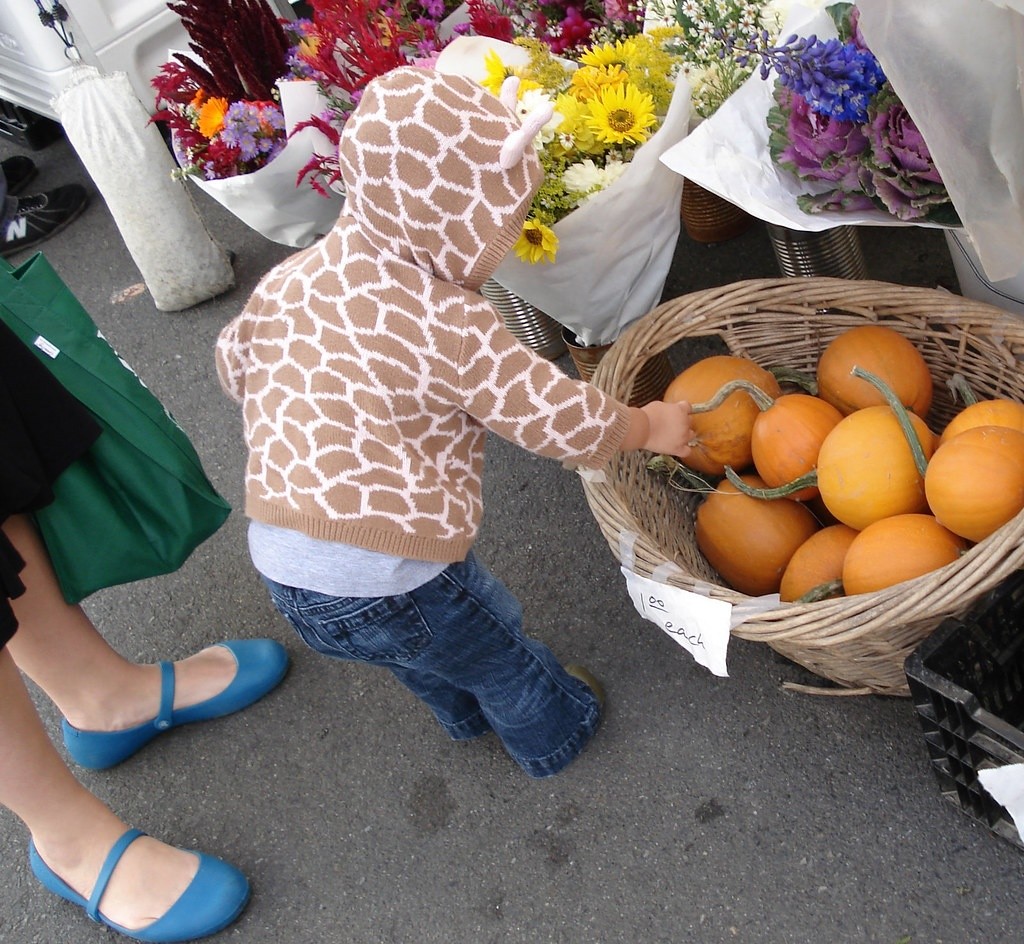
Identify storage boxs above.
[904,572,1024,853]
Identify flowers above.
[284,0,471,198]
[709,0,956,220]
[465,0,645,61]
[144,0,346,248]
[478,24,683,268]
[628,0,790,119]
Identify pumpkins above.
[661,324,1024,603]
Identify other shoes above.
[28,830,251,943]
[565,664,604,706]
[64,636,288,770]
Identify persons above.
[0,156,91,258]
[0,321,293,944]
[214,63,700,776]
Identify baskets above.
[578,275,1023,698]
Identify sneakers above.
[0,183,87,256]
[0,156,36,195]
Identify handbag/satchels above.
[0,250,230,605]
